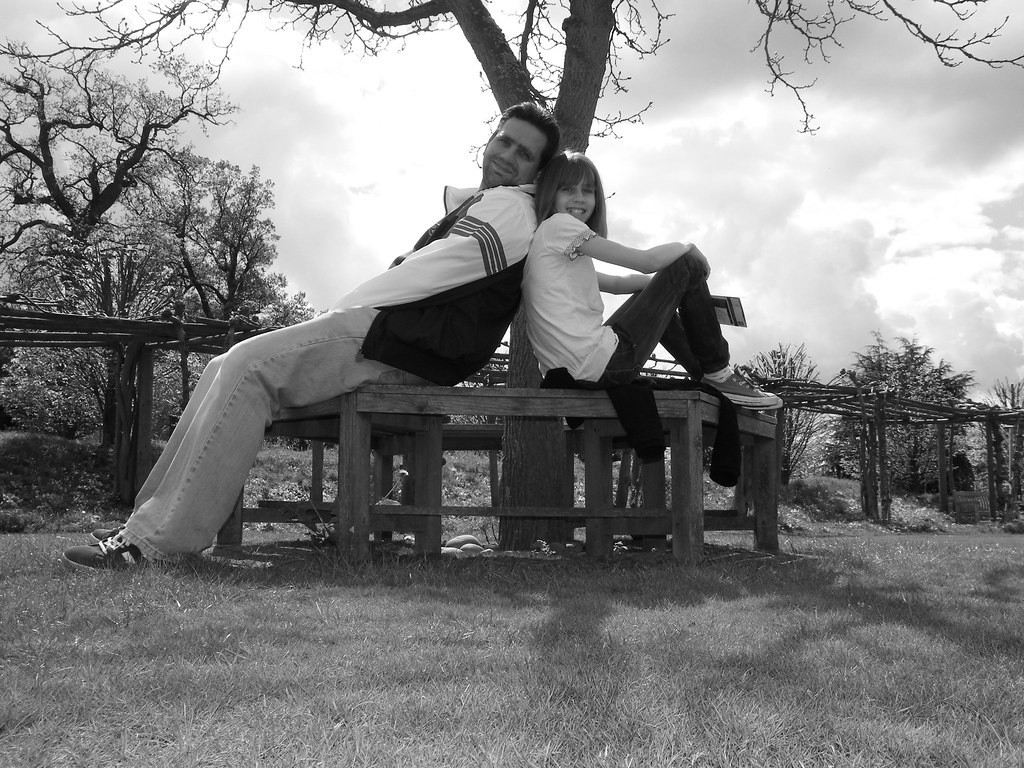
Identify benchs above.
[952,491,990,524]
[214,382,775,565]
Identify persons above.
[524,153,783,410]
[61,103,561,572]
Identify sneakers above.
[701,363,783,410]
[61,533,148,571]
[90,525,126,542]
